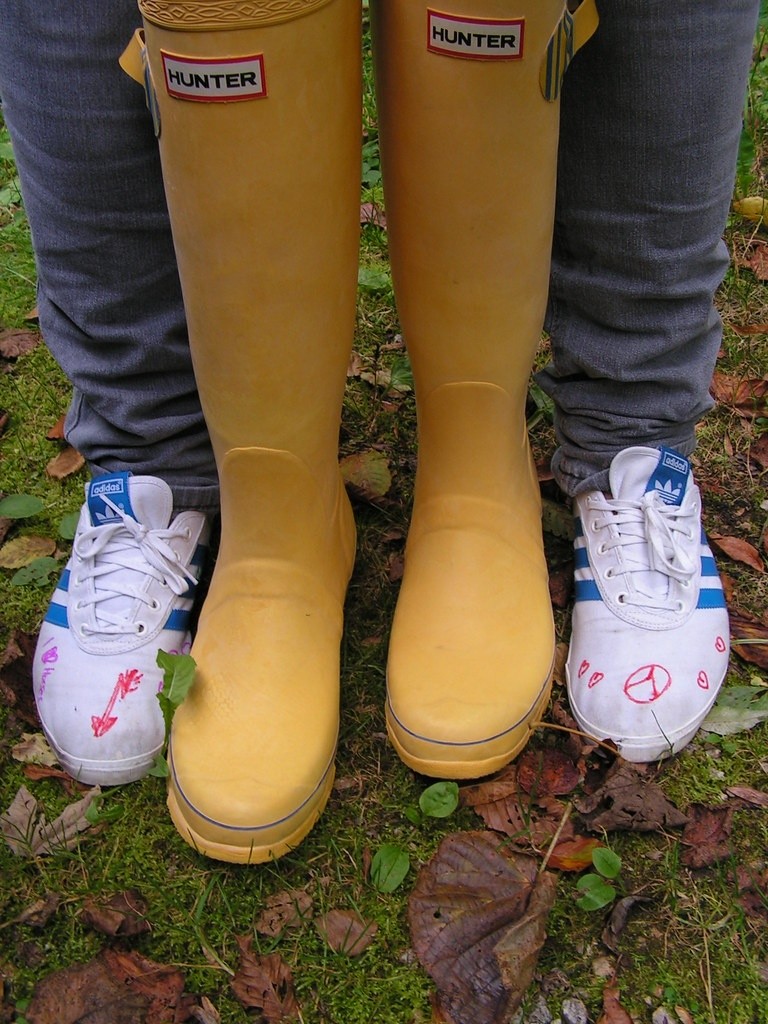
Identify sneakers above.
[565,445,731,763]
[32,472,213,786]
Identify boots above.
[373,0,601,780]
[120,0,362,865]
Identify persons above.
[0,1,730,785]
